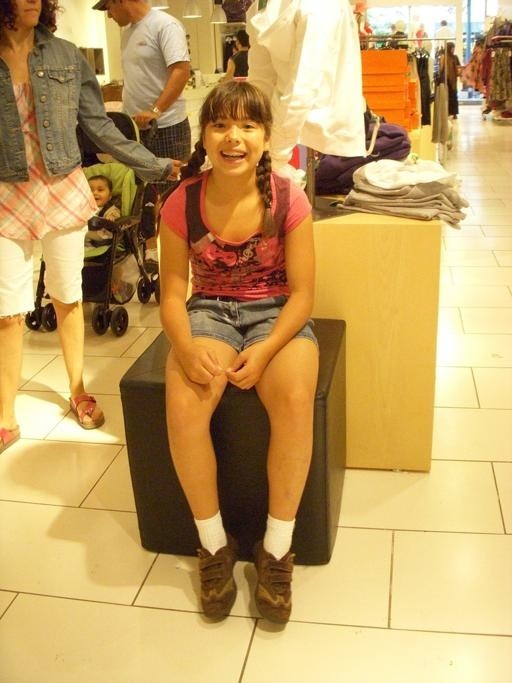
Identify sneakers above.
[254,543,296,623]
[196,533,241,624]
[142,258,160,274]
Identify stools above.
[119,313,346,565]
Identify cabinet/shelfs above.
[312,198,441,473]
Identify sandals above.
[0,425,22,454]
[68,395,105,430]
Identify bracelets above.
[150,106,161,116]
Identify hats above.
[91,0,108,11]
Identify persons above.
[386,20,452,59]
[434,42,461,119]
[84,175,120,246]
[219,30,250,82]
[160,81,320,624]
[92,1,191,272]
[1,1,182,450]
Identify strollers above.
[24,111,161,337]
